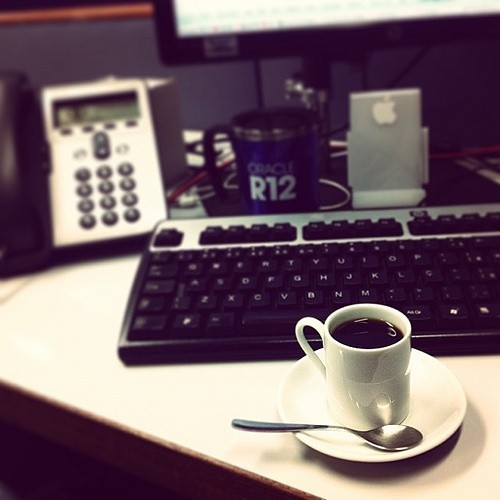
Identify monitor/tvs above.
[150,1,500,68]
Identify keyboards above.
[118,199,500,374]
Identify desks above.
[0,130,499,499]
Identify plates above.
[276,348,467,463]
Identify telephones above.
[0,71,170,266]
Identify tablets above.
[348,87,423,191]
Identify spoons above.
[232,419,423,451]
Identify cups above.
[295,304,411,430]
[203,106,325,213]
